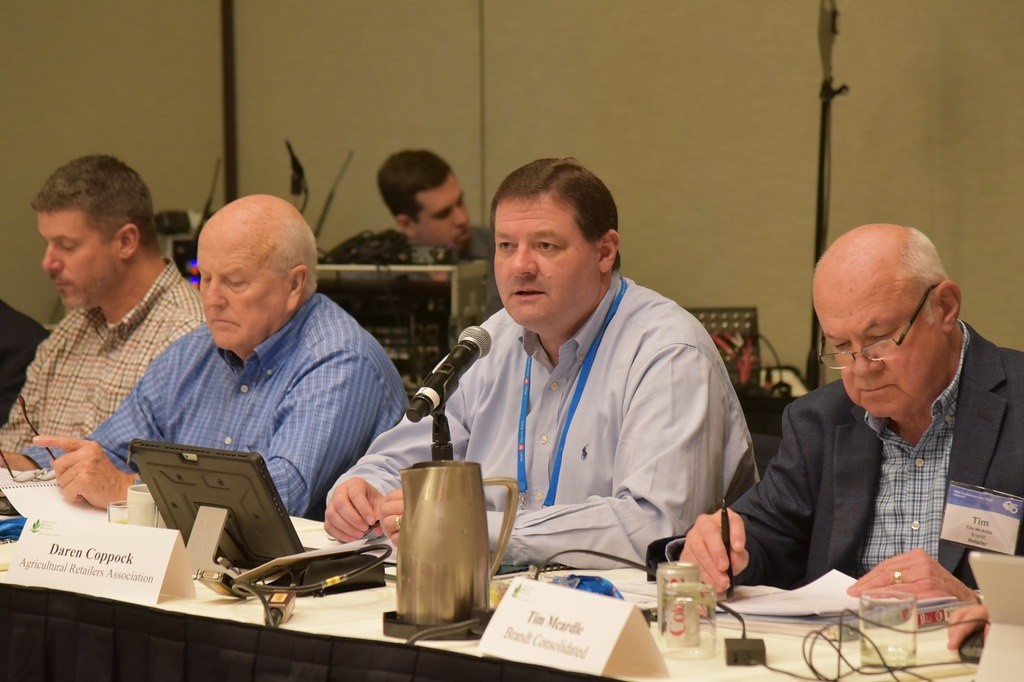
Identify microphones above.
[406,326,492,423]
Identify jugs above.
[396,460,520,626]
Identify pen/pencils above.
[363,519,380,537]
[721,498,734,598]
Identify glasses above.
[0,395,59,483]
[818,282,939,370]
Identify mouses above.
[958,627,986,665]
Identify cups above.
[127,484,158,527]
[108,501,159,527]
[859,590,916,671]
[658,582,718,661]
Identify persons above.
[1,155,206,457]
[377,149,496,267]
[644,220,1024,602]
[0,299,51,429]
[946,605,991,650]
[325,158,762,572]
[0,195,409,518]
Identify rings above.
[894,571,902,584]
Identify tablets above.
[967,551,1024,626]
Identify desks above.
[0,514,978,682]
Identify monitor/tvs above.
[127,437,308,572]
